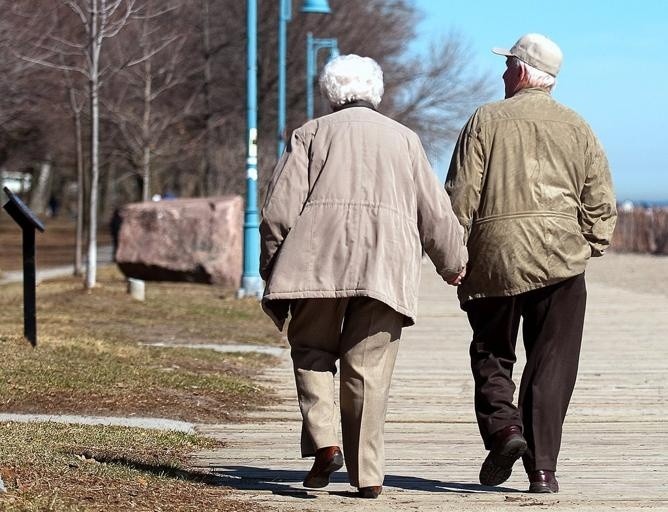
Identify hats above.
[488,31,564,78]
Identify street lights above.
[276,0,339,173]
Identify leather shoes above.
[299,447,346,489]
[526,471,563,495]
[473,423,530,486]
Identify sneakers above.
[359,484,383,501]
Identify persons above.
[48,192,63,217]
[161,183,176,199]
[108,207,124,264]
[258,53,470,499]
[442,31,618,494]
